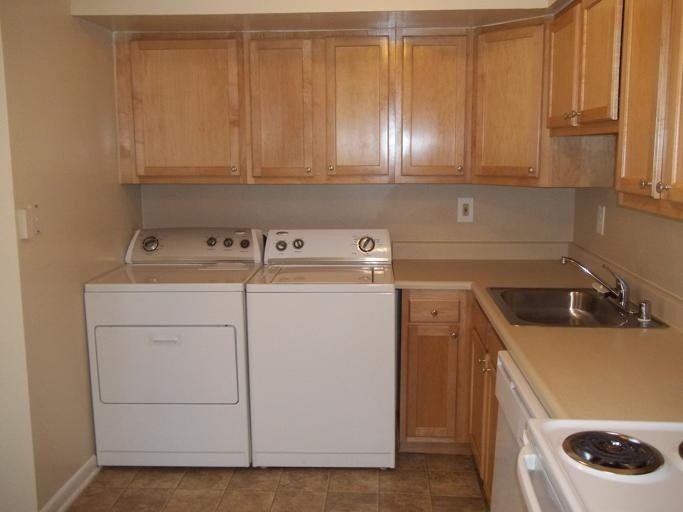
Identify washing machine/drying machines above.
[81,223,264,470]
[243,225,399,472]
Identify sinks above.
[499,287,631,328]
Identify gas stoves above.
[551,426,683,512]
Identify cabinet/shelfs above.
[467,14,616,189]
[392,257,469,455]
[541,1,623,137]
[388,27,473,185]
[108,30,247,187]
[469,290,506,511]
[611,1,683,221]
[241,28,398,186]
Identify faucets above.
[557,253,629,309]
